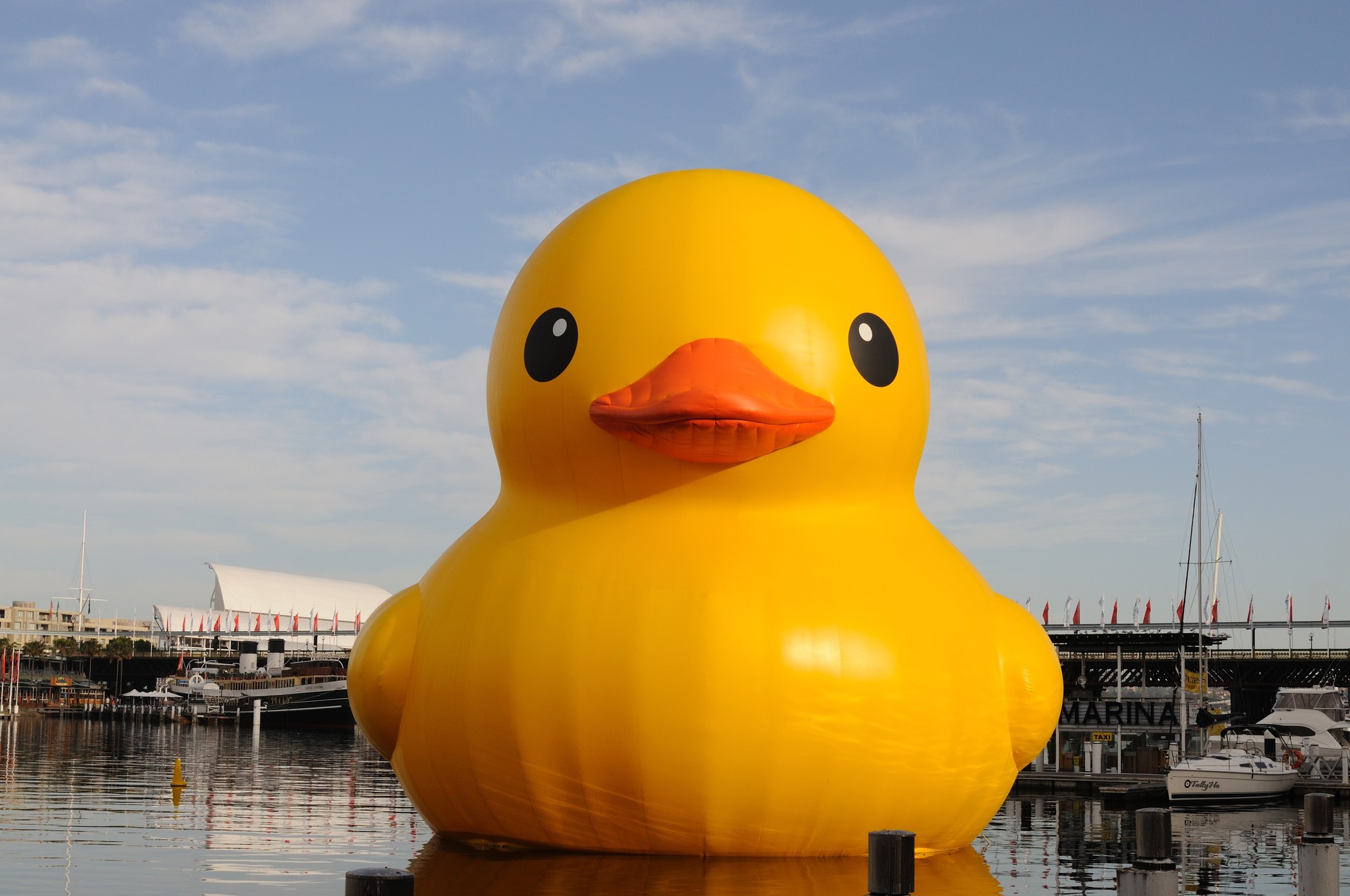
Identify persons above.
[106,697,119,704]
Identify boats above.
[168,639,355,728]
[1187,710,1350,774]
[1165,748,1298,805]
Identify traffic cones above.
[170,758,187,785]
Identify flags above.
[1133,595,1141,627]
[1212,598,1217,623]
[1072,601,1080,634]
[1246,597,1254,630]
[1203,596,1211,625]
[0,648,19,683]
[1098,594,1105,627]
[166,609,360,636]
[1285,594,1292,629]
[1171,595,1183,626]
[1321,595,1330,629]
[1025,595,1031,612]
[1143,600,1151,623]
[49,601,59,619]
[1111,600,1117,624]
[1063,596,1072,627]
[178,651,182,669]
[1043,602,1049,624]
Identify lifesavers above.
[1283,750,1304,770]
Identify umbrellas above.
[121,689,184,706]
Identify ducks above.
[348,166,1064,860]
